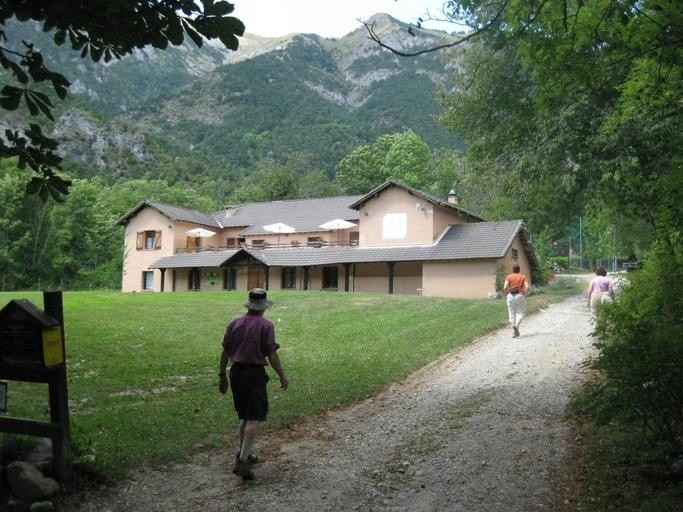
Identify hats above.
[242,287,274,310]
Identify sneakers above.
[513,325,520,339]
[232,458,255,480]
[236,452,258,466]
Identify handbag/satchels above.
[511,288,520,295]
[600,290,613,305]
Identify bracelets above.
[218,372,227,378]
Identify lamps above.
[416,203,425,211]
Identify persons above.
[217,287,290,482]
[585,266,615,330]
[502,261,529,338]
